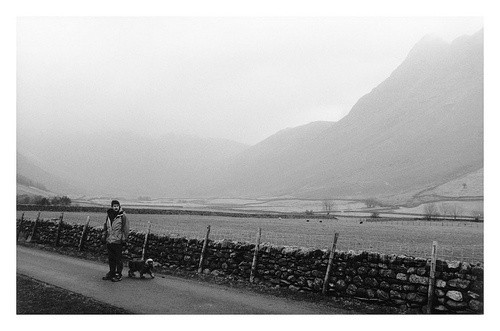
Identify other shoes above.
[103,270,116,280]
[111,272,122,282]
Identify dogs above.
[126,259,154,279]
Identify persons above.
[99,199,130,281]
